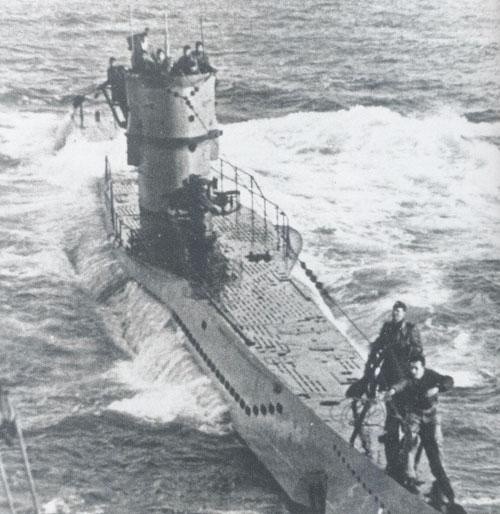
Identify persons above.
[102,24,219,122]
[363,300,426,444]
[379,353,456,512]
